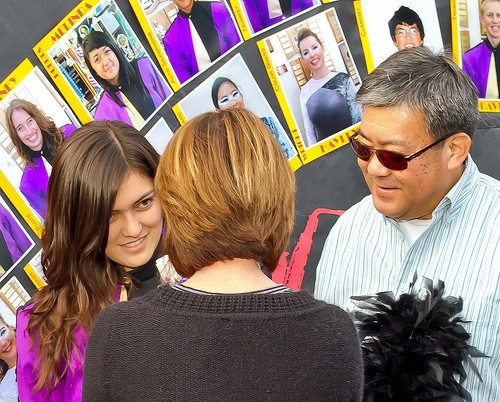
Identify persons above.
[388,5,425,51]
[297,28,362,147]
[243,0,313,33]
[0,203,32,277]
[82,31,171,131]
[16,119,189,402]
[162,0,241,84]
[462,0,500,99]
[82,107,364,402]
[5,99,78,221]
[0,314,16,402]
[314,46,500,402]
[211,77,295,160]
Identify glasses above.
[394,28,421,37]
[349,129,455,172]
